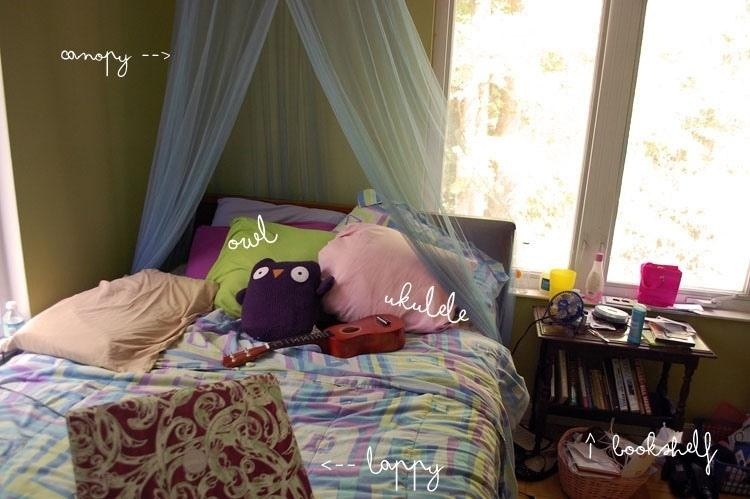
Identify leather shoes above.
[668,444,719,499]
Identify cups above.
[515,271,530,293]
[549,268,577,301]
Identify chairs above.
[65,373,314,499]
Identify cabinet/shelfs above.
[530,305,718,458]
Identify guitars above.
[222,314,405,367]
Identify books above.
[640,317,696,351]
[564,441,621,480]
[543,344,705,414]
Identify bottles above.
[584,254,606,300]
[627,302,647,344]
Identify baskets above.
[557,427,660,498]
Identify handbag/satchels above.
[638,262,682,307]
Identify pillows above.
[0,268,220,374]
[185,197,508,342]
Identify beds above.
[0,197,516,499]
